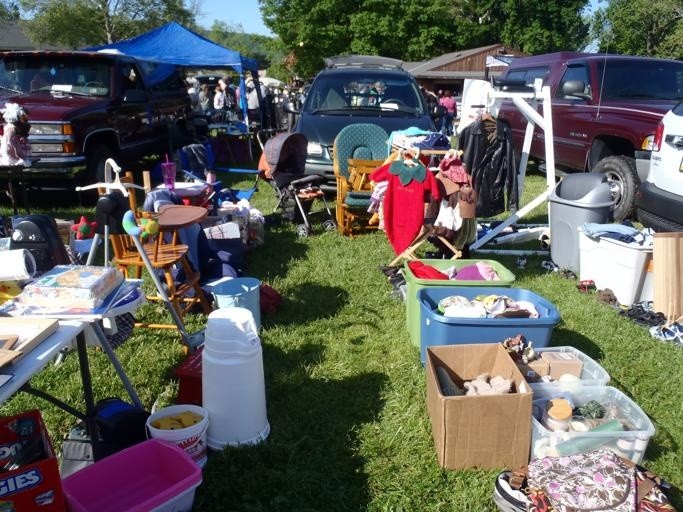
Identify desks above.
[0,287,146,467]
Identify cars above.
[195,75,314,110]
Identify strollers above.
[250,132,336,240]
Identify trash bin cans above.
[547,172,616,280]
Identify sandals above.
[620,304,647,320]
[637,312,665,327]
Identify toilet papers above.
[0,248,38,282]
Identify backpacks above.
[3,214,72,276]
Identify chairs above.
[171,140,264,220]
[97,171,211,353]
[333,123,392,235]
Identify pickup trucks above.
[0,47,190,209]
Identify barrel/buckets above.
[210,276,263,333]
[201,307,271,452]
[144,404,210,469]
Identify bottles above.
[606,405,619,421]
[203,170,216,196]
[542,398,573,431]
[161,162,176,191]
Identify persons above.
[182,73,308,131]
[342,80,389,109]
[418,84,458,136]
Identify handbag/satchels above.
[491,446,677,512]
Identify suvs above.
[292,55,448,197]
[494,52,683,226]
[631,101,683,235]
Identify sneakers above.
[515,256,528,271]
[561,268,617,304]
[648,322,683,348]
[539,260,558,272]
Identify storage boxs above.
[415,287,561,367]
[529,385,656,466]
[576,224,654,307]
[63,438,202,512]
[402,258,516,350]
[0,410,67,512]
[425,342,535,470]
[510,343,611,396]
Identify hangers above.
[399,146,421,168]
[426,150,465,179]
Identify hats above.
[458,185,478,222]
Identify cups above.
[567,414,590,432]
